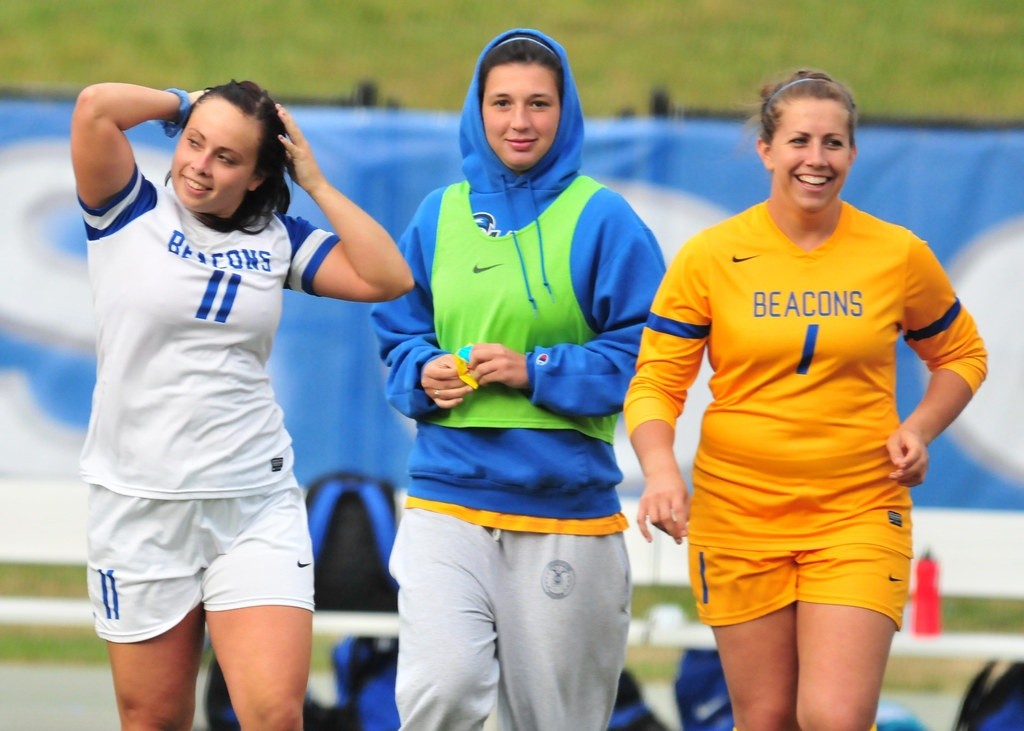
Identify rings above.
[435,389,439,399]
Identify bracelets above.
[159,87,191,138]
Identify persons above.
[623,69,988,731]
[73,79,416,731]
[368,28,664,730]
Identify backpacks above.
[299,474,402,611]
[203,656,312,731]
[676,647,738,731]
[605,672,665,731]
[952,657,1024,731]
[332,637,401,731]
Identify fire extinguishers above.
[912,546,941,636]
